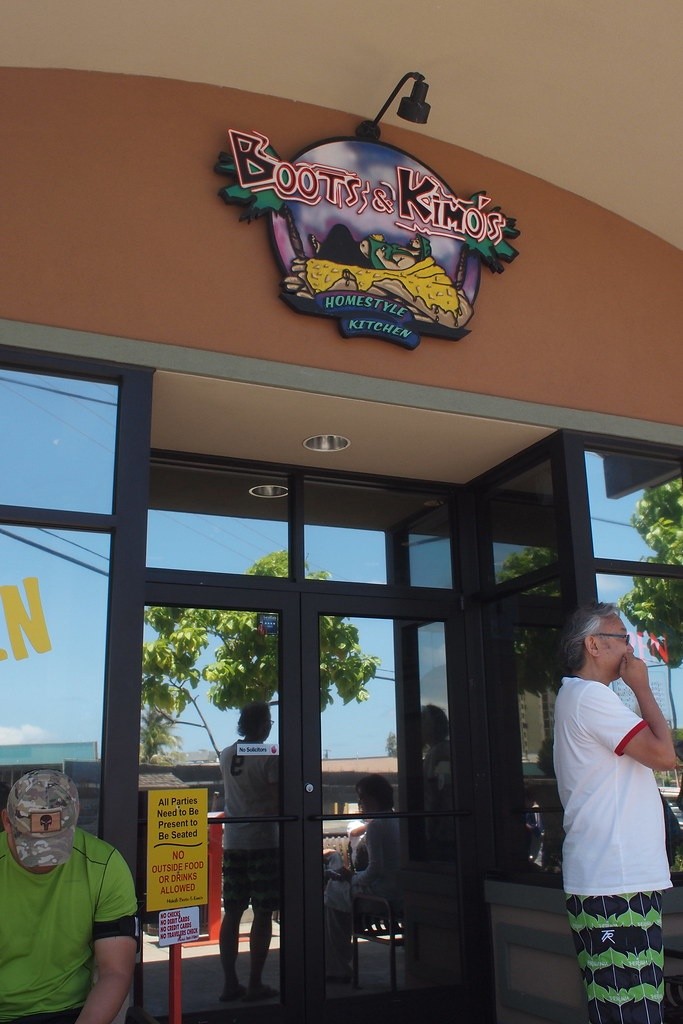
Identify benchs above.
[351,894,405,992]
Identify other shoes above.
[325,973,353,984]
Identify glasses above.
[263,720,274,725]
[582,633,631,646]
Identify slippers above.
[242,985,280,1002]
[219,984,247,1002]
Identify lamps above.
[355,73,430,140]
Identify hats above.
[7,769,80,867]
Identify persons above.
[553,601,675,1024]
[0,771,139,1024]
[219,702,284,999]
[423,703,451,840]
[326,774,400,981]
